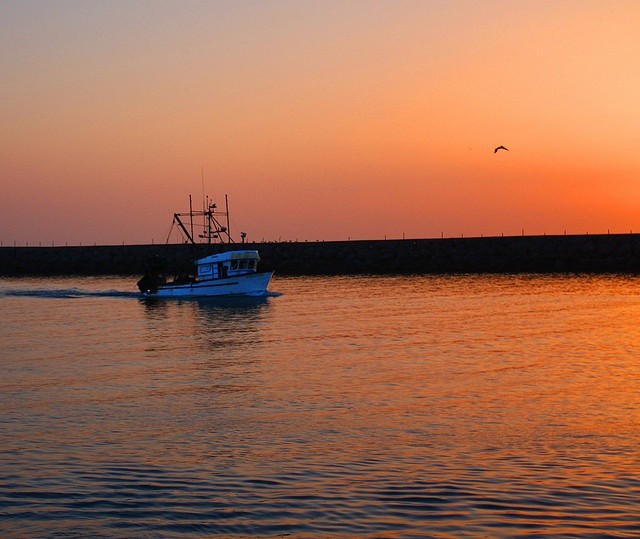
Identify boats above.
[135,189,277,303]
